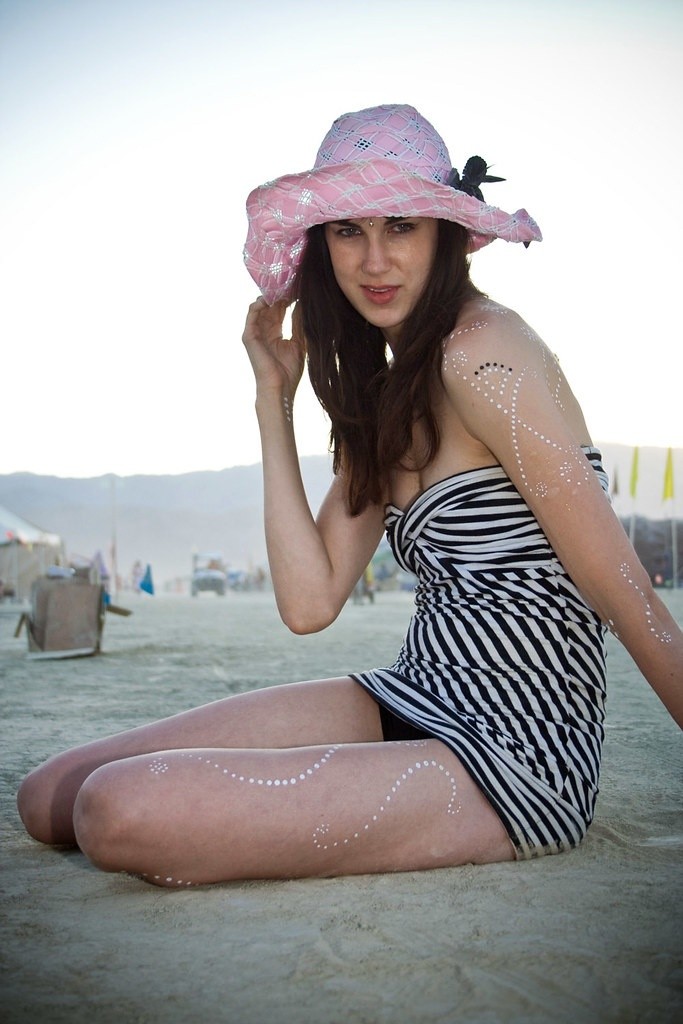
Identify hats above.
[243,103,544,305]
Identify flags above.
[630,447,637,496]
[660,449,673,502]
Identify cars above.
[187,549,239,599]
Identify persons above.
[209,561,218,568]
[133,560,142,594]
[18,103,682,887]
[365,563,374,591]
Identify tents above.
[1,511,61,602]
